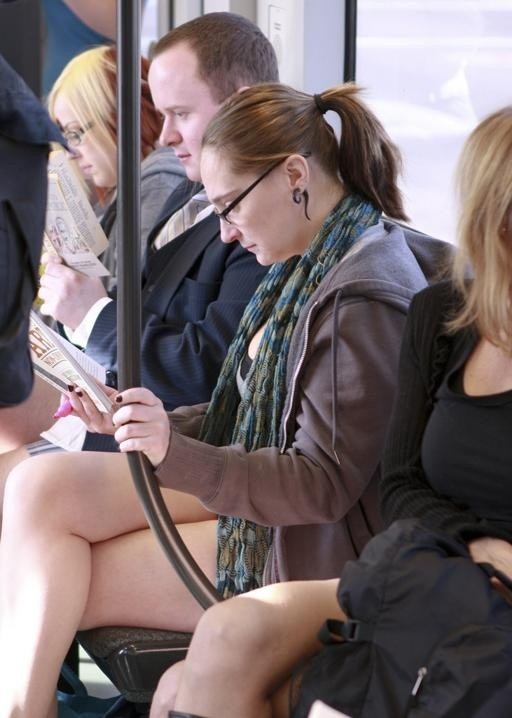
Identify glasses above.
[215,150,312,224]
[65,122,92,148]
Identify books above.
[41,149,113,279]
[27,310,114,414]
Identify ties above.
[153,188,211,250]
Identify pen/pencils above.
[50,402,74,421]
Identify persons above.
[47,43,189,296]
[146,104,508,718]
[42,0,150,151]
[0,13,282,502]
[1,83,463,718]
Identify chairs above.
[74,216,460,702]
